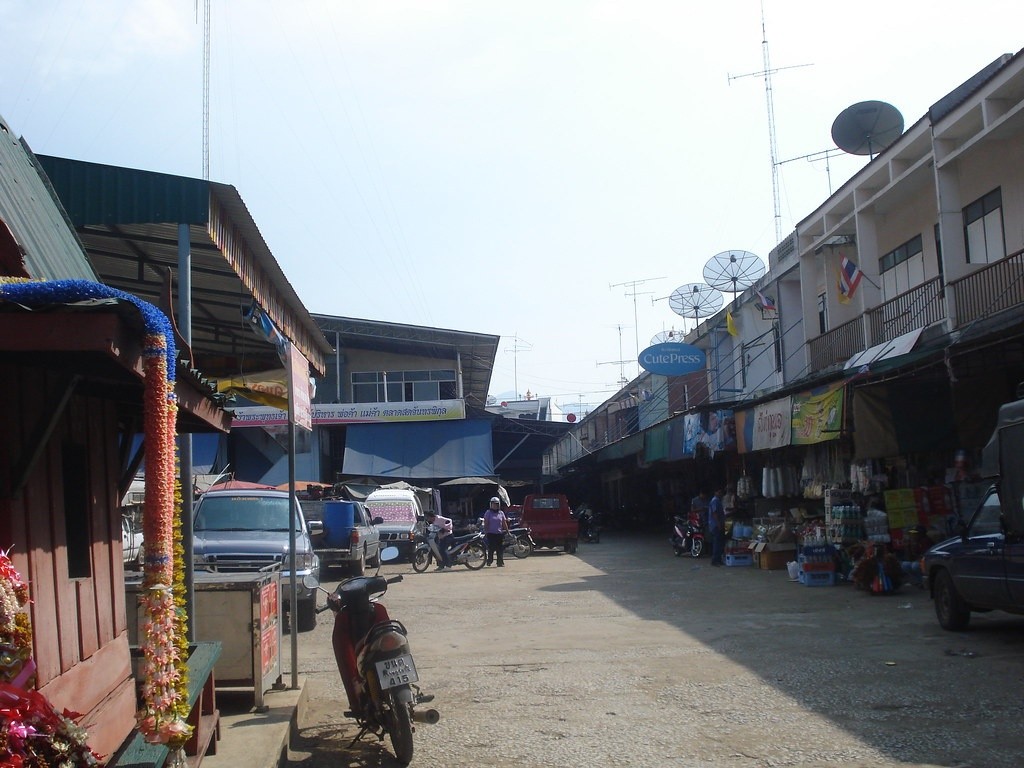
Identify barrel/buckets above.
[322,500,355,548]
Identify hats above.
[908,524,928,534]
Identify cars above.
[193,491,322,633]
[366,490,428,557]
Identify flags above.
[727,312,737,337]
[753,288,768,307]
[840,254,863,299]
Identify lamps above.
[754,303,764,311]
[764,295,775,307]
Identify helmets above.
[490,497,501,510]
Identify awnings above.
[728,306,1024,413]
[555,400,752,474]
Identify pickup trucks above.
[296,500,385,578]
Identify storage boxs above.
[725,554,753,567]
[746,542,797,571]
[883,485,926,550]
[824,487,853,542]
[802,571,834,586]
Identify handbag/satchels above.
[872,564,893,593]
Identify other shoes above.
[711,561,721,567]
[496,563,505,567]
[442,566,452,572]
[434,567,444,572]
[487,563,490,566]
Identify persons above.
[725,488,736,512]
[692,488,709,557]
[709,487,726,567]
[484,497,512,567]
[427,512,454,572]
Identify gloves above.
[439,528,446,534]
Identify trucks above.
[919,400,1024,633]
[519,494,579,554]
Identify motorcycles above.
[668,513,704,559]
[469,517,536,560]
[303,545,440,766]
[412,521,488,574]
[571,502,600,545]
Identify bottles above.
[831,505,862,519]
